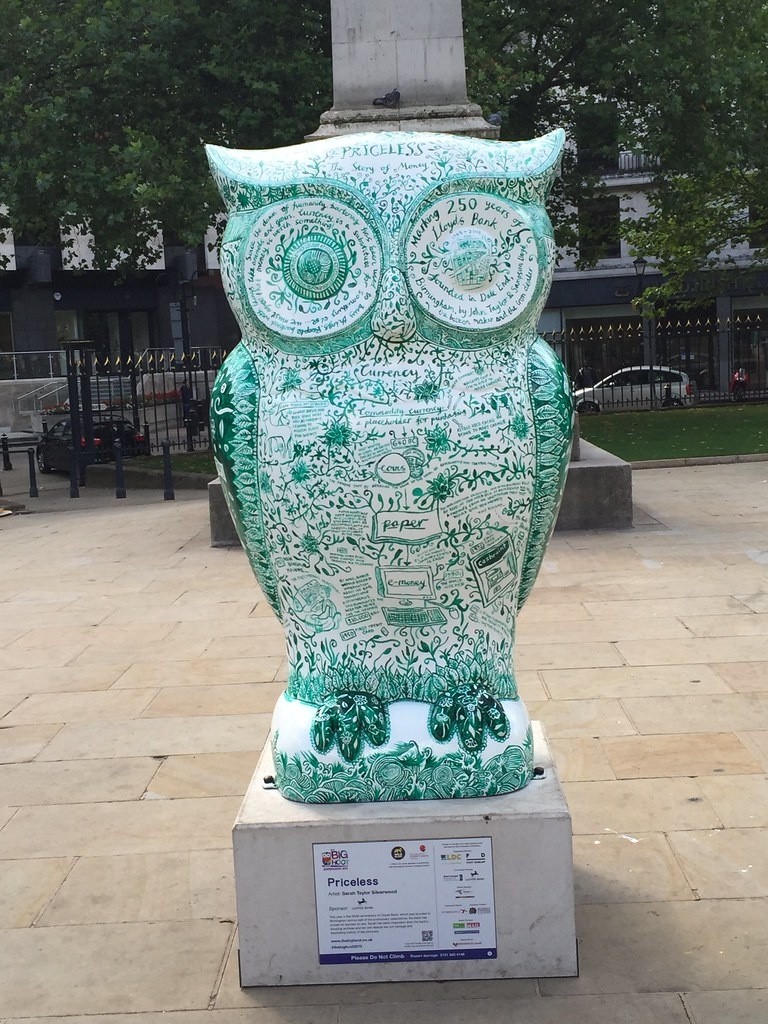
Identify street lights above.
[167,270,214,436]
[632,256,646,364]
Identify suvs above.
[572,365,700,414]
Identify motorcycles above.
[729,369,749,403]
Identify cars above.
[36,415,151,486]
